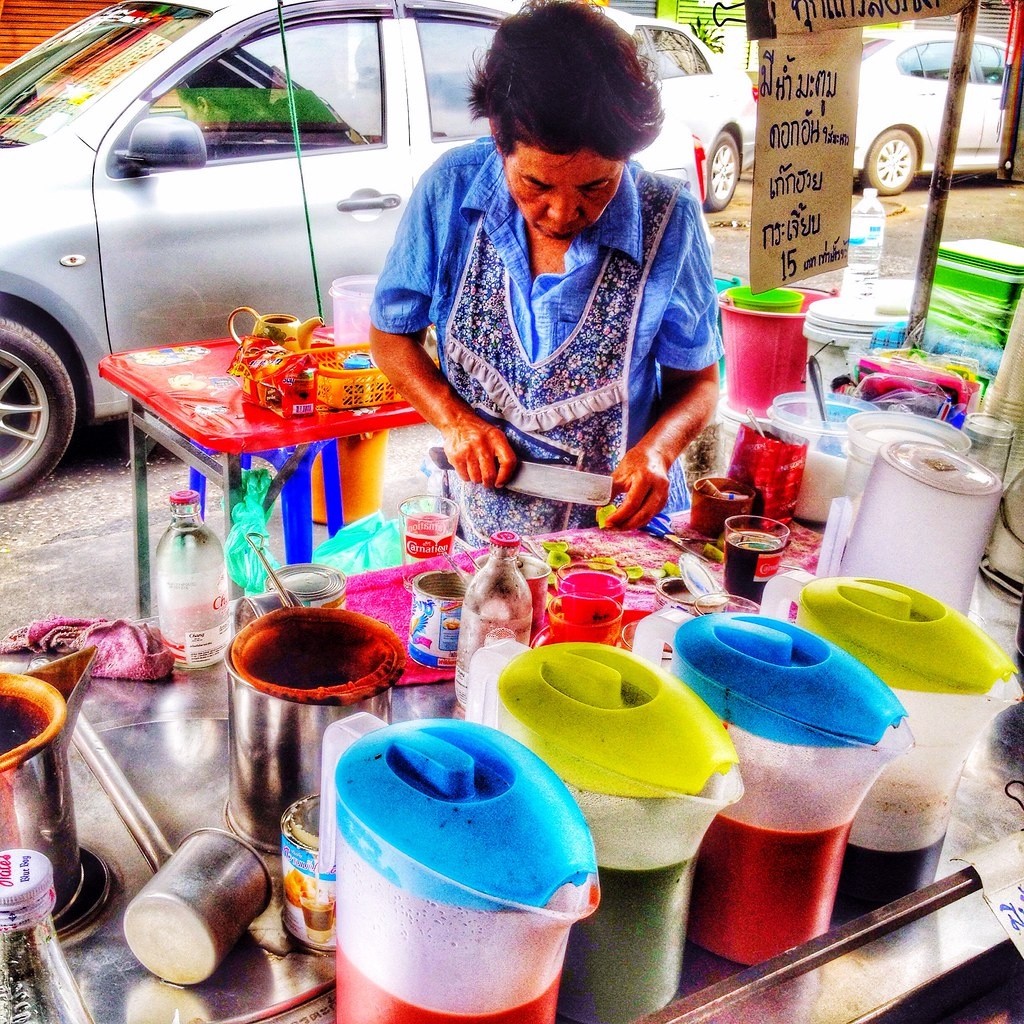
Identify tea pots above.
[227,306,325,352]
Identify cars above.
[603,7,759,213]
[853,28,1009,197]
[0,0,514,507]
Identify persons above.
[175,62,267,160]
[370,0,727,557]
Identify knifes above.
[427,447,613,507]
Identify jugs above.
[841,411,972,500]
[815,442,1002,616]
[318,572,1024,1024]
[0,645,97,932]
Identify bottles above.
[454,531,534,710]
[838,188,884,311]
[156,489,232,668]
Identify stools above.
[189,439,344,565]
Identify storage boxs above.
[928,237,1024,348]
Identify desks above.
[98,338,427,619]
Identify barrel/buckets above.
[280,794,336,953]
[708,275,909,476]
[406,572,473,669]
[265,563,347,611]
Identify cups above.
[302,890,335,942]
[767,392,883,524]
[124,828,273,986]
[474,553,672,670]
[961,285,1024,494]
[398,495,458,593]
[693,478,755,538]
[694,515,789,618]
[328,274,377,350]
